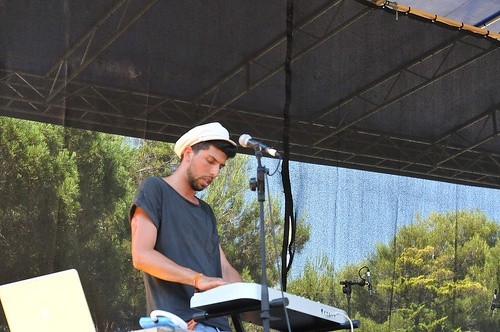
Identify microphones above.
[239,133,280,159]
[488,289,497,317]
[365,268,372,297]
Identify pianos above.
[189,281,349,332]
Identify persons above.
[129,121,243,332]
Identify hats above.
[173,121,236,160]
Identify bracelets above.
[194,272,205,293]
